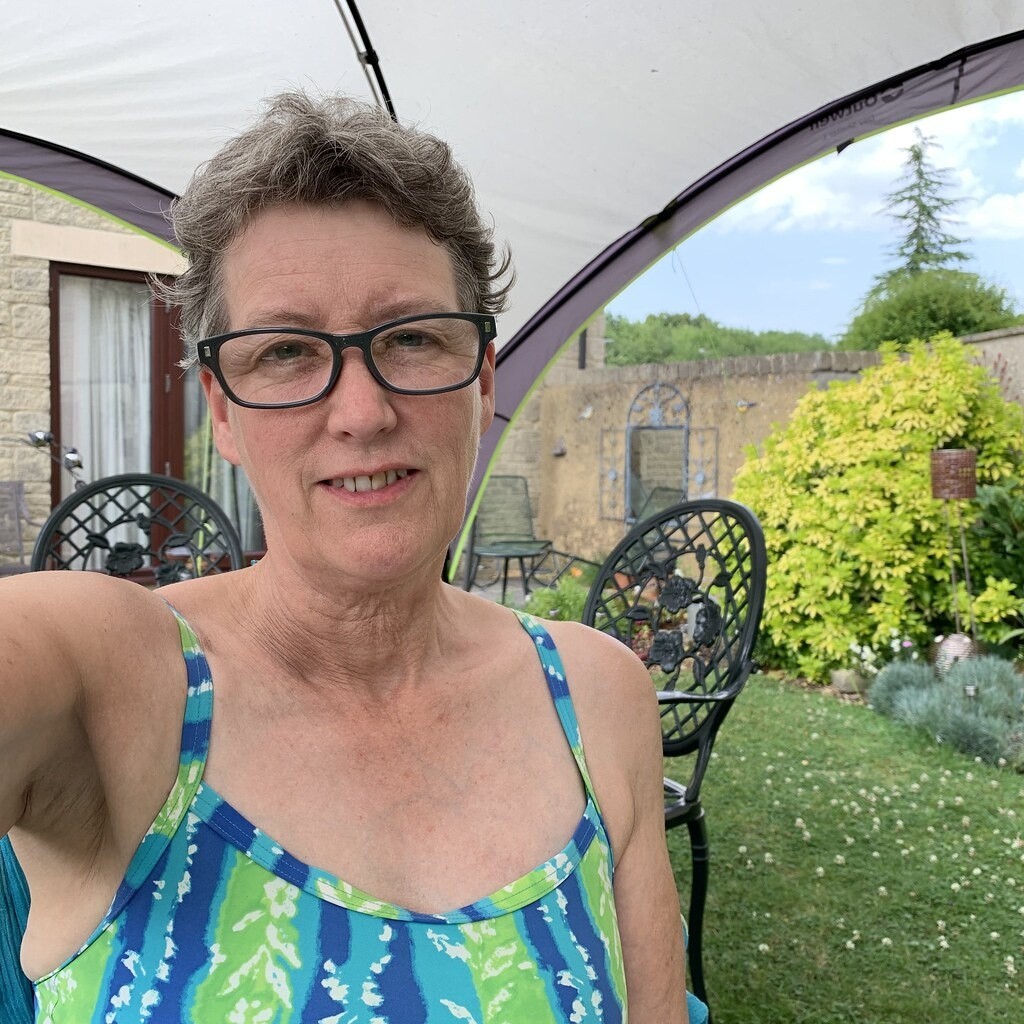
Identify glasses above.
[197,314,497,409]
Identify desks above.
[471,544,546,605]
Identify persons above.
[0,95,689,1024]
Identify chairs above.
[467,475,560,588]
[30,474,246,589]
[580,498,766,1024]
